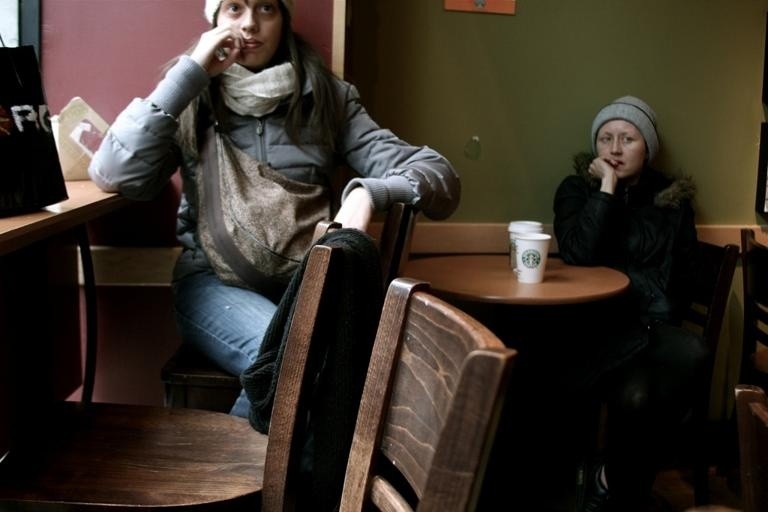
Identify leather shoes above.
[586,465,608,509]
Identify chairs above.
[674,235,740,462]
[335,274,513,512]
[731,226,767,506]
[4,222,342,506]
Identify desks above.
[402,253,629,314]
[0,178,173,466]
[158,197,422,425]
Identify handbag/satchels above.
[196,133,330,287]
[0,34,68,216]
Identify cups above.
[508,220,544,271]
[515,232,552,284]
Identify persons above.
[89,0,462,423]
[552,96,703,507]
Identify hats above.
[203,0,293,29]
[592,96,660,162]
